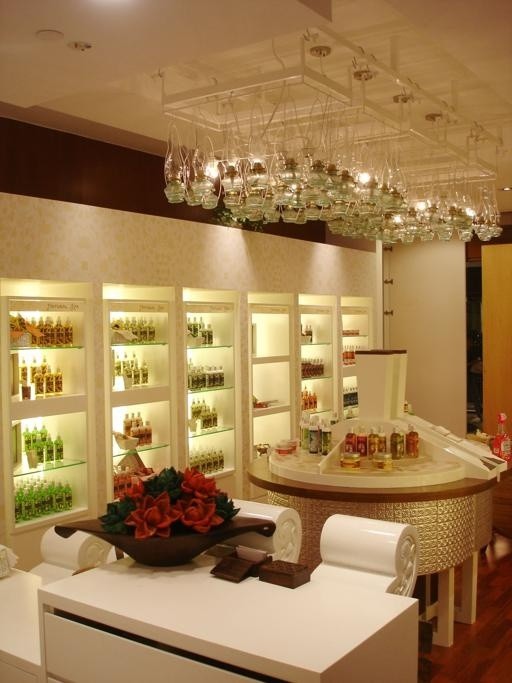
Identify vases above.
[52,517,275,567]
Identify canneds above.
[340,451,360,470]
[372,452,392,472]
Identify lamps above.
[158,24,505,243]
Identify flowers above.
[98,467,240,542]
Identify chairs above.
[30,526,115,585]
[229,498,303,565]
[309,512,418,595]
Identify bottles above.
[187,317,213,345]
[111,315,156,498]
[345,425,420,458]
[187,357,224,390]
[188,400,225,475]
[299,410,331,454]
[404,399,408,413]
[276,437,299,455]
[306,323,313,343]
[332,409,340,426]
[301,324,305,336]
[342,330,359,406]
[8,313,74,524]
[346,406,354,417]
[491,413,512,463]
[371,453,393,470]
[300,357,326,410]
[341,452,360,468]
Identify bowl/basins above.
[302,336,312,344]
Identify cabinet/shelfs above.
[248,302,294,460]
[299,304,333,441]
[0,295,99,535]
[183,305,237,478]
[0,499,419,683]
[104,298,172,475]
[340,305,371,411]
